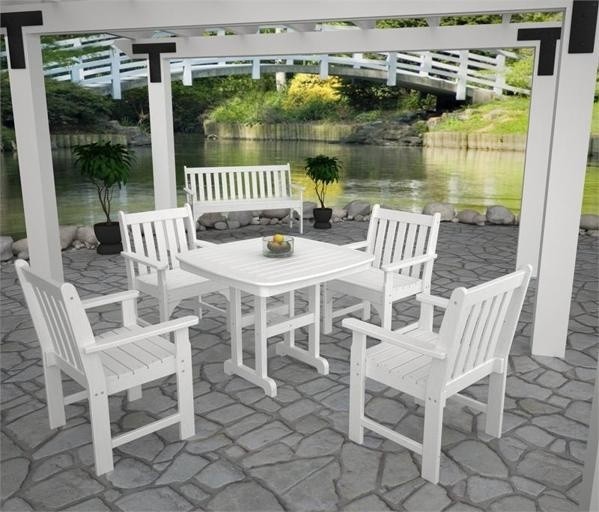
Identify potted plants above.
[71,133,135,255]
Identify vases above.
[306,146,340,228]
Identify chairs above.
[322,204,443,341]
[342,264,533,483]
[13,259,200,478]
[115,203,244,345]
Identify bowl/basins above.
[261,234,294,258]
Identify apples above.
[267,233,291,251]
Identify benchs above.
[181,162,309,235]
